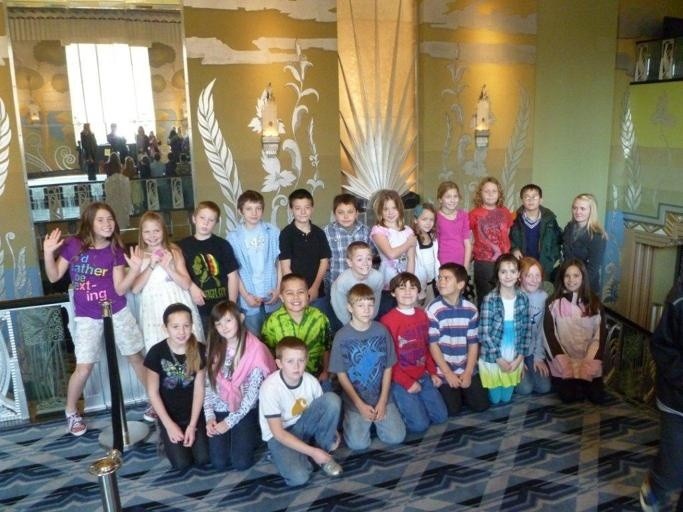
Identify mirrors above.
[2,2,195,299]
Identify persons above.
[259,189,407,487]
[510,183,607,403]
[370,177,532,431]
[636,265,683,512]
[76,120,191,232]
[44,202,157,436]
[132,191,280,471]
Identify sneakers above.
[322,462,344,477]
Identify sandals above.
[65,409,87,437]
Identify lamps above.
[259,82,281,157]
[475,85,490,150]
